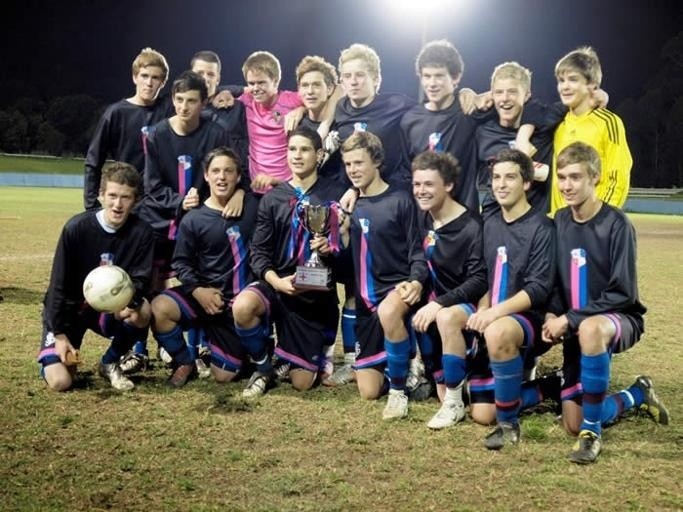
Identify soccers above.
[83,265,134,314]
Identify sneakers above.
[406,352,422,391]
[381,384,410,424]
[428,380,468,429]
[541,367,564,420]
[158,341,357,398]
[118,350,149,373]
[484,424,520,452]
[97,357,134,391]
[632,373,671,426]
[569,427,605,465]
